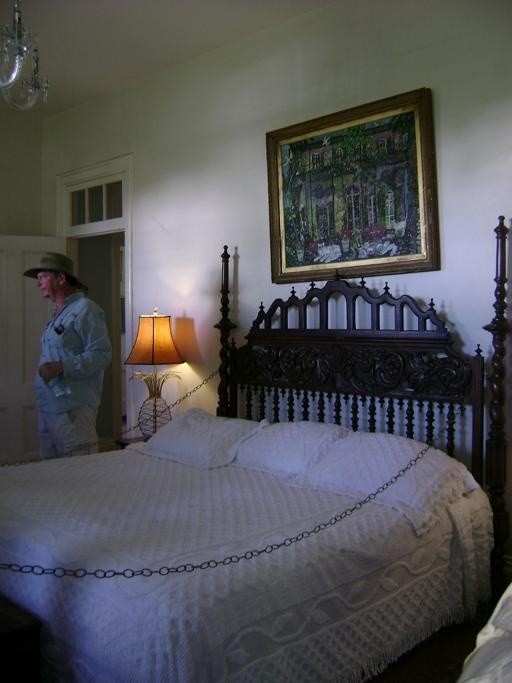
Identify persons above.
[22,251,113,461]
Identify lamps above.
[0,0,49,110]
[123,307,186,439]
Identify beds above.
[0,216,512,683]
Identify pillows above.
[125,407,269,470]
[230,421,342,478]
[282,430,480,537]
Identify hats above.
[24,252,83,289]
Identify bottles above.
[48,374,71,398]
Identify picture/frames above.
[266,87,441,284]
